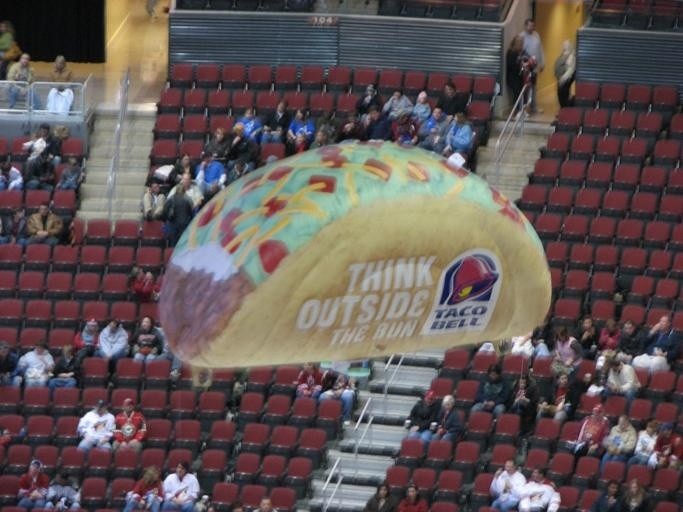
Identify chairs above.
[1,0,683,512]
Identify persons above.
[0,22,77,245]
[143,80,479,239]
[515,21,543,114]
[361,312,682,511]
[505,33,530,118]
[553,39,578,109]
[1,267,361,509]
